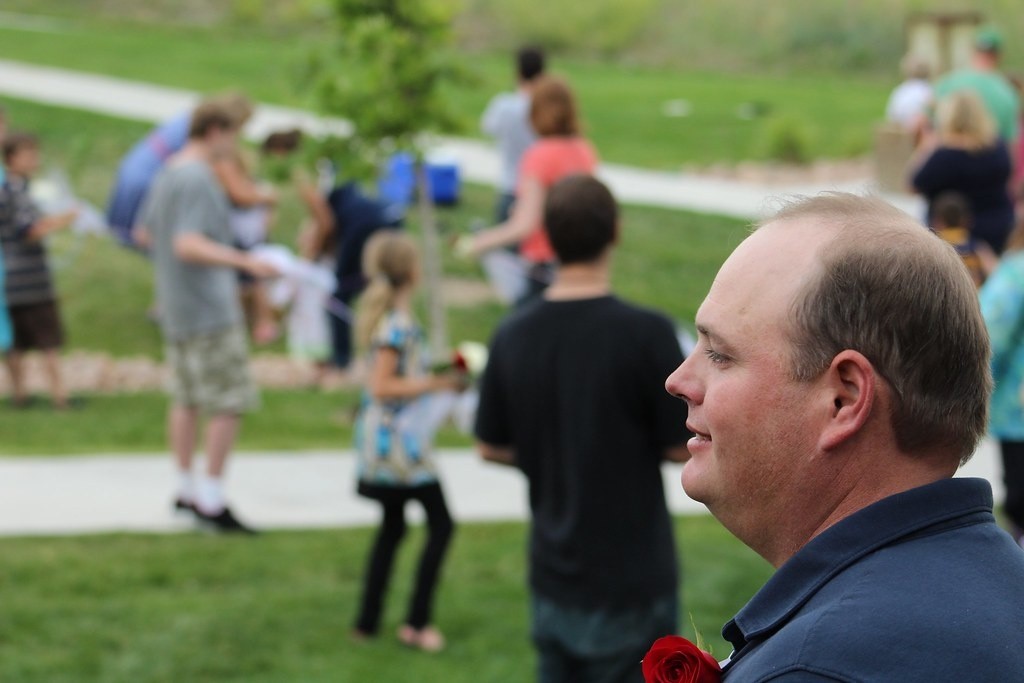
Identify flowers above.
[640,611,726,683]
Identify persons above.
[101,87,280,333]
[343,228,469,653]
[467,168,704,683]
[451,70,602,303]
[655,192,1024,683]
[906,28,1018,152]
[886,52,935,143]
[265,120,399,380]
[973,228,1023,546]
[0,123,98,415]
[130,96,280,541]
[475,43,559,265]
[901,83,1014,283]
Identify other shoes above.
[24,393,44,410]
[176,501,255,534]
[68,394,96,410]
[398,624,446,651]
